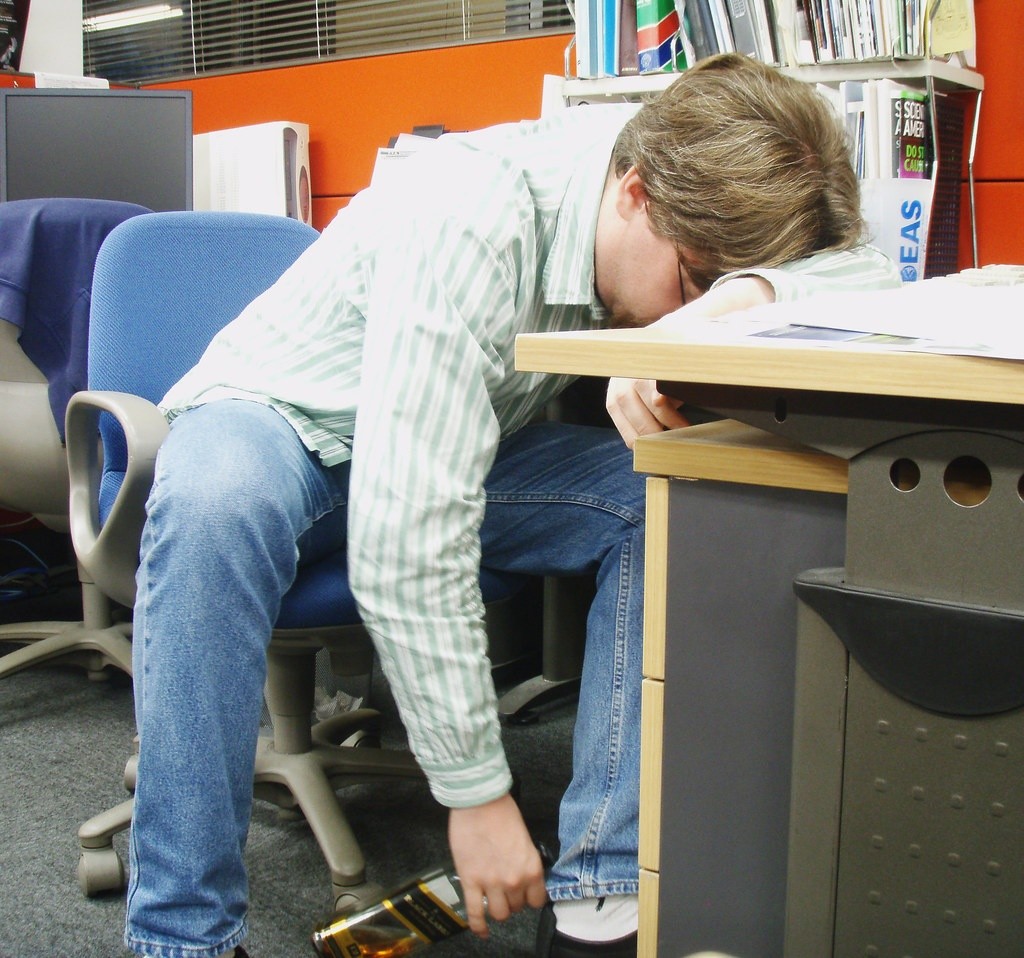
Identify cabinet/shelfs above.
[541,0,985,285]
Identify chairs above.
[64,209,531,914]
[0,197,162,681]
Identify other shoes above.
[535,902,640,958]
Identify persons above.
[123,49,867,958]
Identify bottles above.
[311,842,555,958]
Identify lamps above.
[80,4,185,33]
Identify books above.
[569,0,932,177]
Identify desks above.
[515,310,1024,958]
